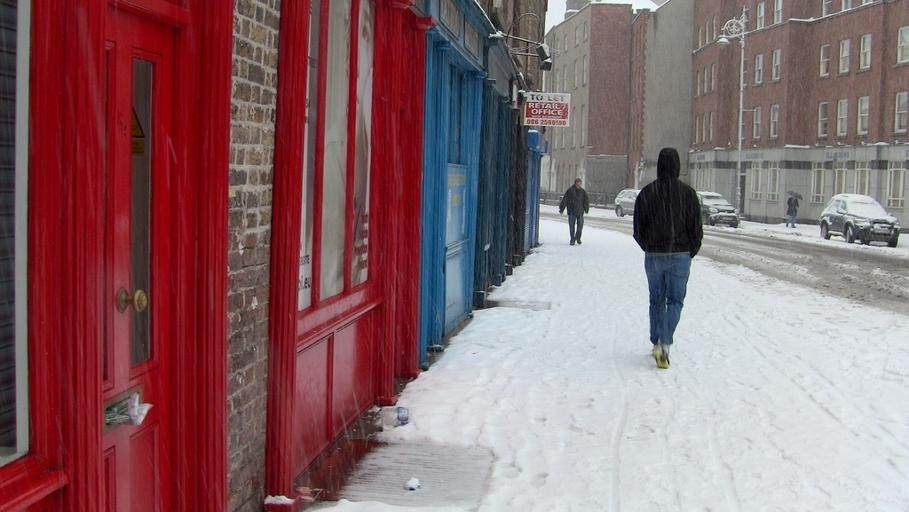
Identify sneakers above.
[569,239,582,246]
[652,344,671,371]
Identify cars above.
[819,194,900,247]
[615,189,641,217]
[695,192,738,228]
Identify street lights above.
[717,17,746,218]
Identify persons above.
[633,147,704,369]
[786,195,800,228]
[558,179,590,246]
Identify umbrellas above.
[786,191,803,200]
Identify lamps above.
[511,52,551,71]
[489,32,551,61]
